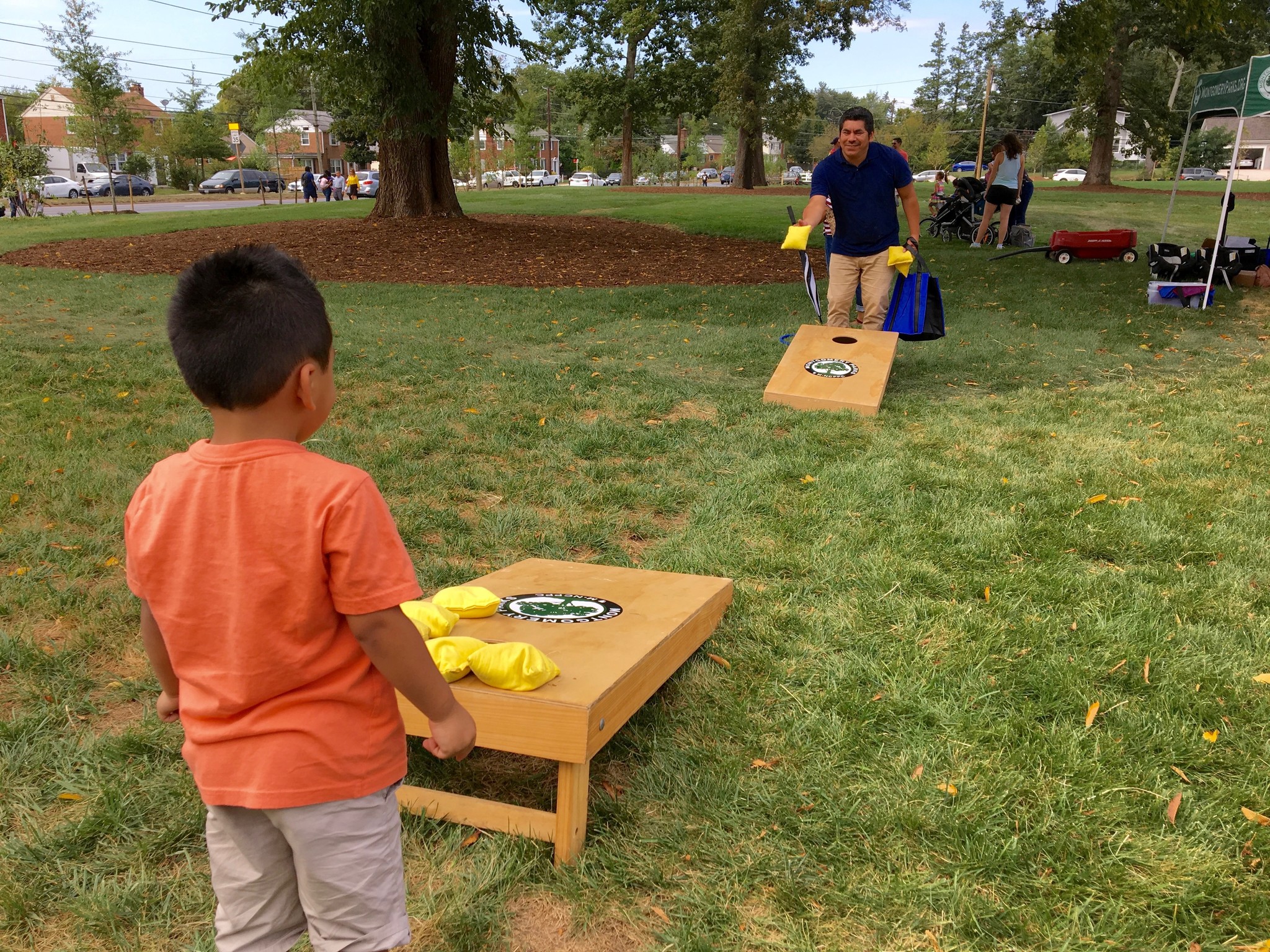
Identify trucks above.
[19,143,116,186]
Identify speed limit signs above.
[230,129,240,144]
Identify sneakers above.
[932,213,937,217]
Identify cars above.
[1212,159,1232,169]
[287,173,336,193]
[952,161,989,172]
[1236,156,1254,169]
[1052,169,1087,181]
[912,169,946,183]
[605,173,622,186]
[636,171,690,185]
[2,175,81,199]
[452,170,525,189]
[79,174,155,198]
[697,168,718,180]
[568,171,606,186]
[789,166,804,173]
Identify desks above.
[393,558,733,872]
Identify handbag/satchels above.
[883,236,946,342]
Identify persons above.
[122,242,477,952]
[794,105,922,336]
[303,164,318,204]
[6,178,53,217]
[970,134,1034,251]
[702,171,709,187]
[319,168,360,202]
[928,171,949,218]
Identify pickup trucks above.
[523,170,560,187]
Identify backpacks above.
[1010,223,1036,248]
[301,172,310,187]
[8,182,19,201]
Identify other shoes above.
[996,246,1005,250]
[970,243,981,248]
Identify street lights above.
[695,123,718,171]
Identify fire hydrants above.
[188,181,194,192]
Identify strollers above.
[918,176,1012,246]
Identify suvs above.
[344,170,380,200]
[262,171,286,193]
[198,168,270,194]
[719,166,735,185]
[1180,168,1226,181]
[782,169,802,185]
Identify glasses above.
[892,143,897,145]
[350,171,352,172]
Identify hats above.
[830,137,839,145]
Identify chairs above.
[1195,247,1242,286]
[1146,243,1194,283]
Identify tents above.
[1161,54,1270,310]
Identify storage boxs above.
[1219,244,1260,271]
[1147,281,1200,309]
